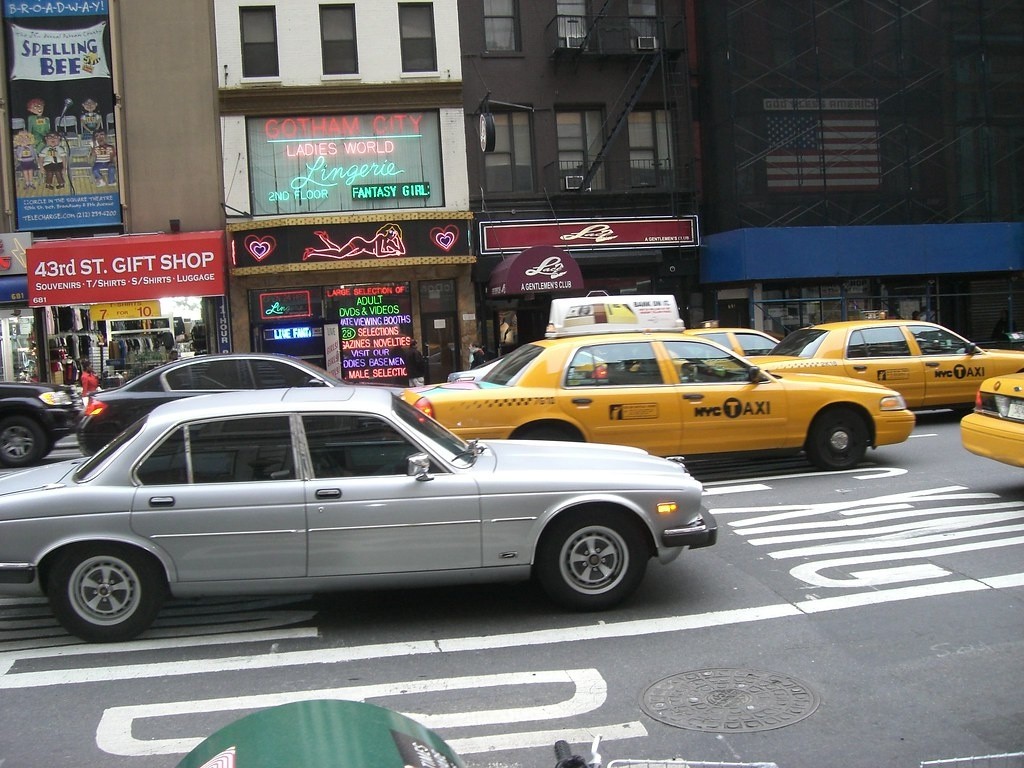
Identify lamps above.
[170,219,180,232]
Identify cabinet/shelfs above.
[106,312,175,347]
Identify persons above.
[991,310,1017,349]
[404,338,425,387]
[72,358,100,425]
[912,310,921,320]
[468,342,486,370]
[170,350,178,361]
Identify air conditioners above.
[567,36,588,49]
[565,176,591,190]
[638,36,657,50]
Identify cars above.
[399,292,916,475]
[0,381,86,469]
[0,388,724,644]
[759,317,1024,415]
[675,327,782,367]
[75,352,394,457]
[956,368,1024,471]
